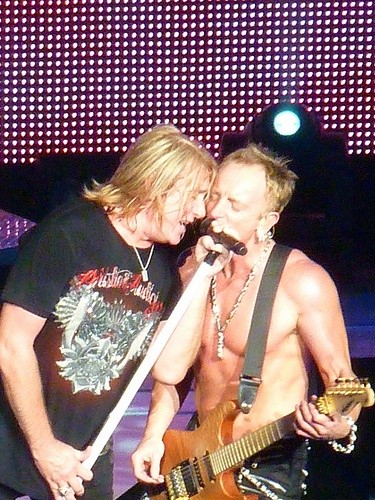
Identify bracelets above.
[327,416,358,455]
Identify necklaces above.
[124,228,156,282]
[209,232,272,358]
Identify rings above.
[58,486,70,496]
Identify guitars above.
[136,376,375,500]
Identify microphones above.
[201,217,248,256]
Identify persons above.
[131,143,365,500]
[1,123,235,499]
